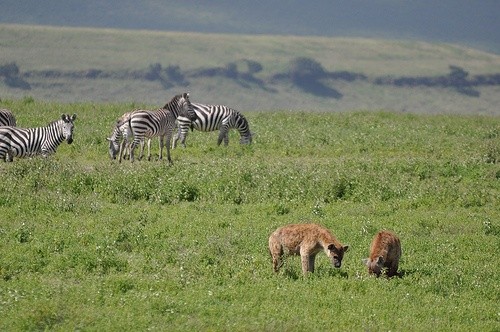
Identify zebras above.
[107,92,252,166]
[0,108,78,164]
